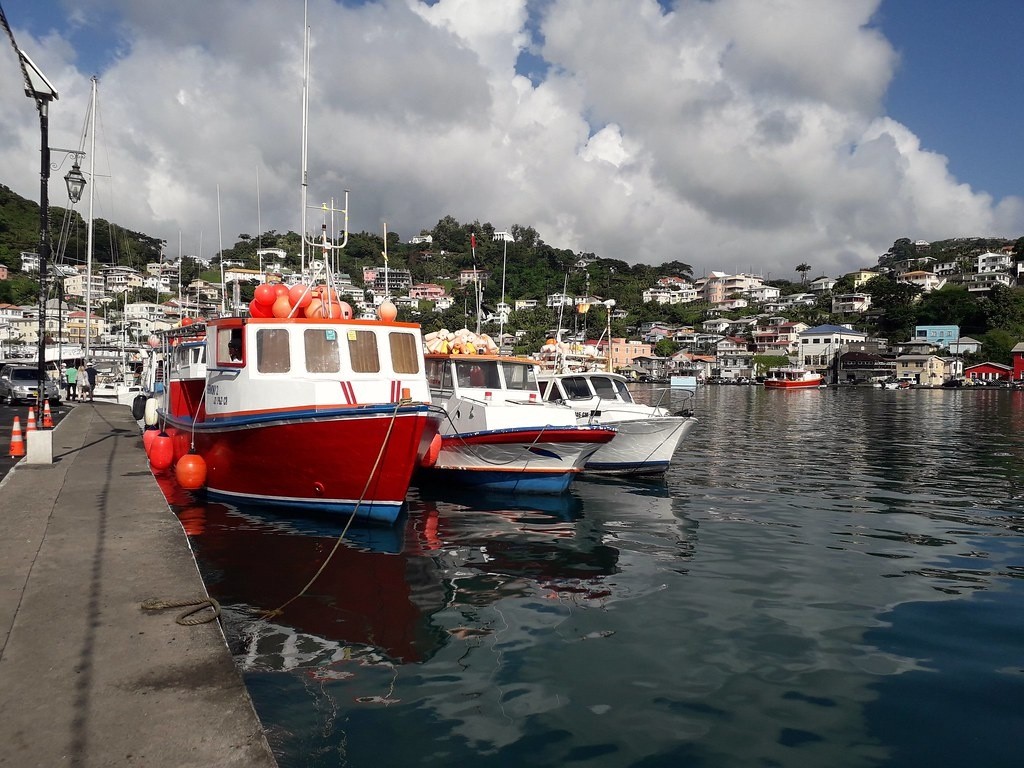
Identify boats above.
[93,0,446,525]
[764,366,824,389]
[413,240,619,495]
[511,274,699,476]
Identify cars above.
[942,378,986,387]
[730,374,757,384]
[0,365,59,405]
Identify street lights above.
[19,50,87,430]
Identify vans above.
[893,377,917,385]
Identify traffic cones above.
[24,407,38,441]
[43,400,55,428]
[4,416,25,457]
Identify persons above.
[75,366,90,403]
[65,363,78,401]
[84,362,97,403]
